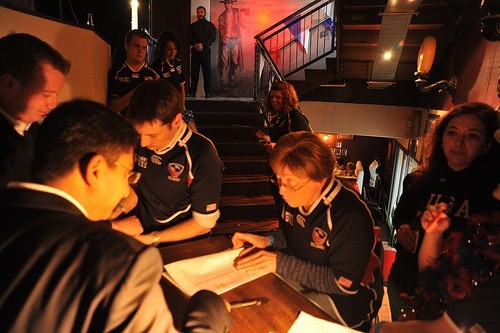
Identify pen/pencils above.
[424,206,452,234]
[230,298,268,308]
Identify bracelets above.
[118,204,125,212]
[183,106,185,111]
[151,231,160,246]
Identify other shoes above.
[186,93,197,97]
[205,94,211,98]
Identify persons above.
[108,79,221,245]
[334,158,344,175]
[149,31,185,111]
[107,30,164,118]
[387,101,500,333]
[231,131,385,333]
[0,98,232,333]
[255,81,311,226]
[0,32,71,188]
[187,5,217,97]
[344,162,356,176]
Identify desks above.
[159,234,338,333]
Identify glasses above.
[270,174,316,192]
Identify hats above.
[219,0,237,4]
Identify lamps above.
[415,35,457,97]
[480,0,500,42]
